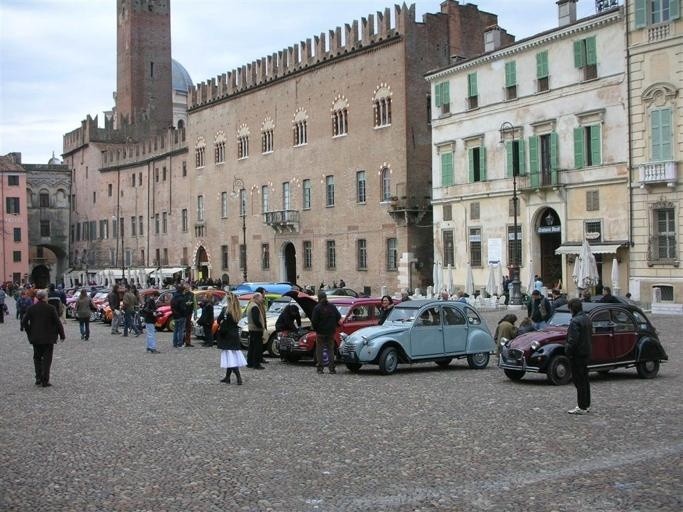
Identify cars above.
[64,280,358,360]
[336,297,491,376]
[272,296,401,366]
[587,295,646,329]
[495,302,669,385]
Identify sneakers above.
[317,367,323,374]
[328,368,336,373]
[568,406,590,414]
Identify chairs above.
[431,292,508,314]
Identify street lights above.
[230,177,247,283]
[496,122,524,305]
[111,205,125,280]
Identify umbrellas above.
[572,257,581,295]
[611,259,620,296]
[436,257,535,297]
[577,237,599,297]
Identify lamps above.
[544,208,555,227]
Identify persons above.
[455,290,467,317]
[378,295,395,326]
[492,275,622,357]
[399,289,413,303]
[441,291,453,315]
[565,298,593,414]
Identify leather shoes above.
[221,376,230,383]
[238,377,242,384]
